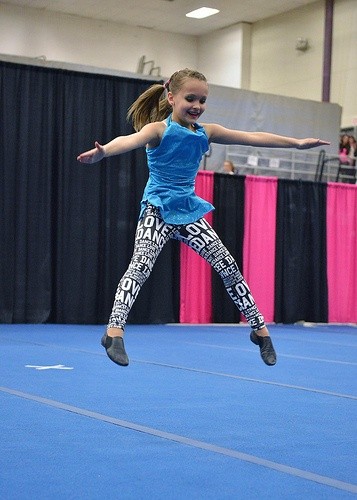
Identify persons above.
[335,135,357,184]
[222,160,235,176]
[77,68,331,366]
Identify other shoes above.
[101,331,130,366]
[249,330,277,366]
